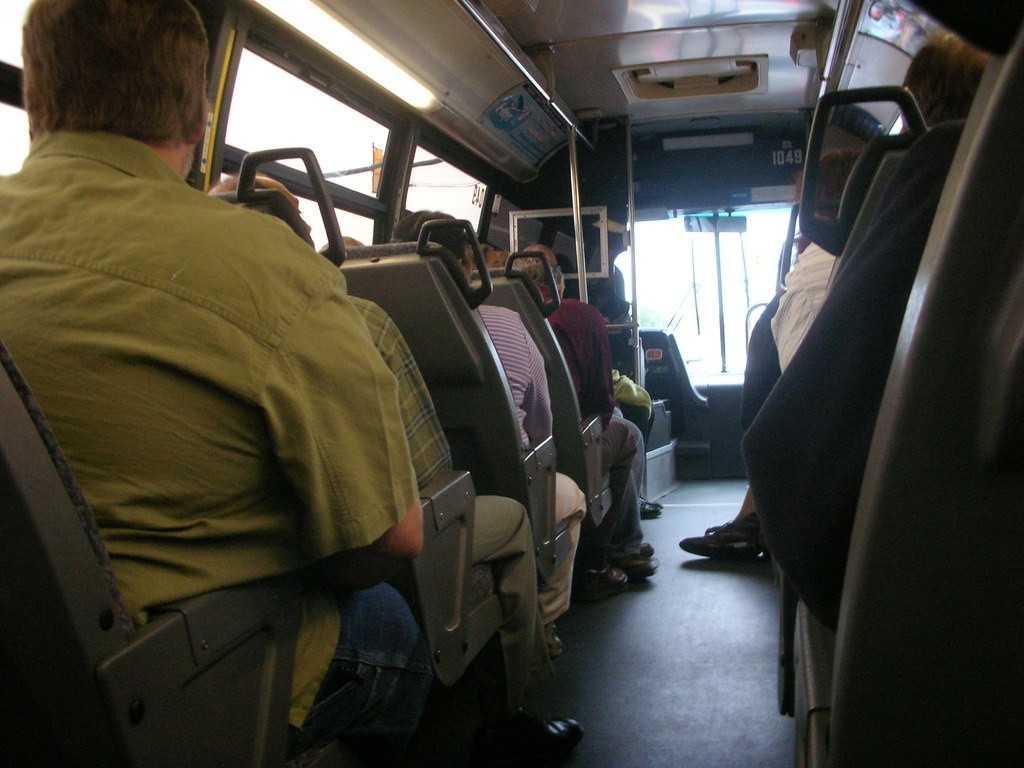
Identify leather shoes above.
[471,704,584,768]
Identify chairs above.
[777,0,1024,768]
[211,149,518,688]
[340,219,572,581]
[637,330,710,410]
[468,252,613,528]
[0,347,306,768]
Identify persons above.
[0,0,664,768]
[680,33,984,559]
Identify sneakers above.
[678,512,763,558]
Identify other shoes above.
[640,543,654,558]
[547,635,563,655]
[638,497,664,518]
[588,564,629,586]
[619,556,654,577]
